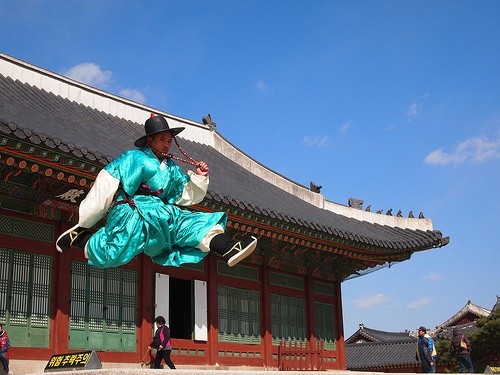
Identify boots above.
[209,233,257,267]
[56,222,94,252]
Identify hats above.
[134,116,186,147]
[416,326,426,331]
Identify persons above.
[0,324,12,375]
[71,114,257,268]
[451,334,473,372]
[148,316,176,369]
[420,338,433,373]
[416,326,436,372]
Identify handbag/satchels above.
[460,335,467,349]
